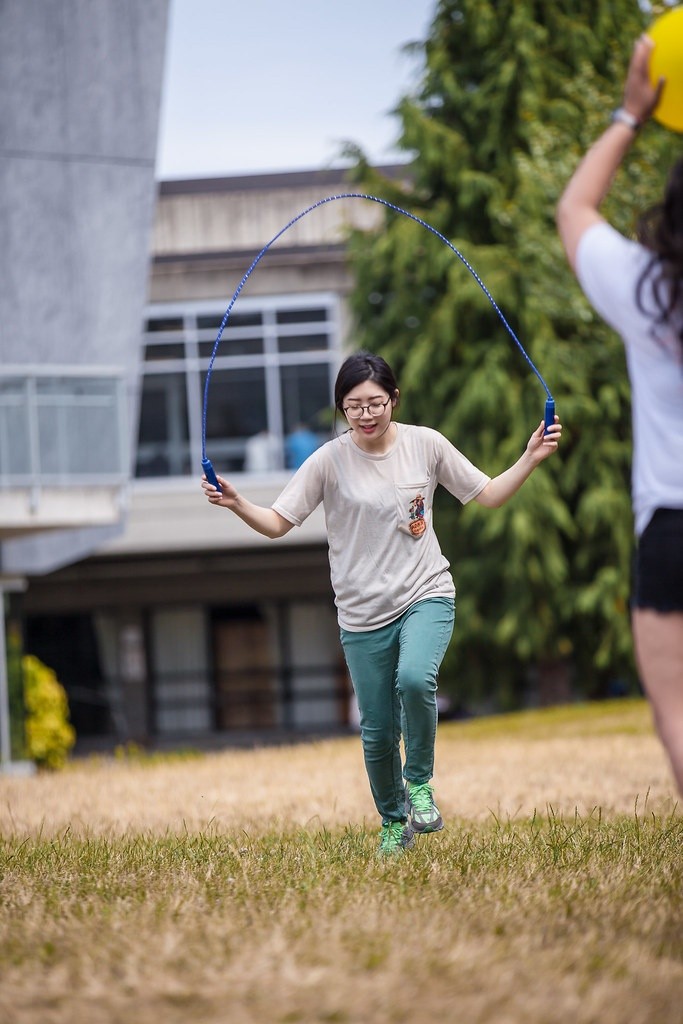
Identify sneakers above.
[403,779,443,834]
[379,820,415,855]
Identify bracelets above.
[611,106,639,130]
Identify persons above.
[202,346,565,855]
[552,29,683,793]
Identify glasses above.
[342,395,393,420]
